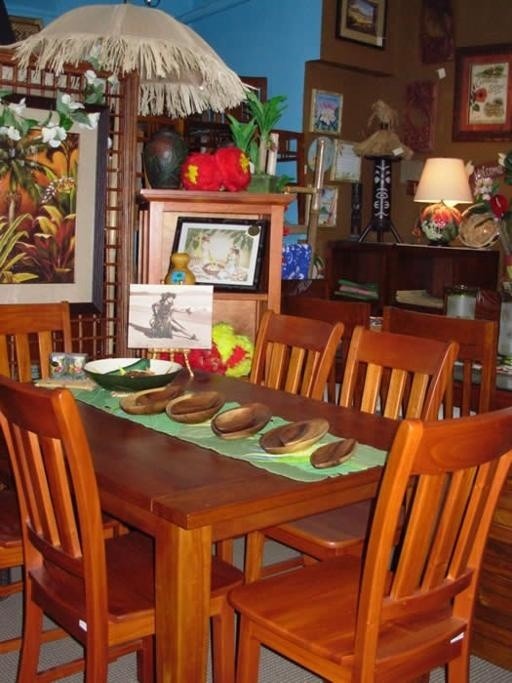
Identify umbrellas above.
[1,0,248,118]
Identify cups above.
[66,352,88,382]
[445,282,479,322]
[49,351,68,381]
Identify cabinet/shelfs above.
[316,235,505,348]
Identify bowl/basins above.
[81,357,184,394]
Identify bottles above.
[165,253,196,286]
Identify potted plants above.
[222,91,284,193]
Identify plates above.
[457,201,500,249]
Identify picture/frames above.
[308,86,344,136]
[327,139,365,182]
[160,216,271,296]
[0,90,111,308]
[304,183,339,229]
[335,1,391,48]
[450,42,511,143]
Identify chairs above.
[226,403,512,681]
[0,369,243,680]
[240,328,465,587]
[218,309,348,575]
[1,296,147,664]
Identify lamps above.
[410,154,476,239]
